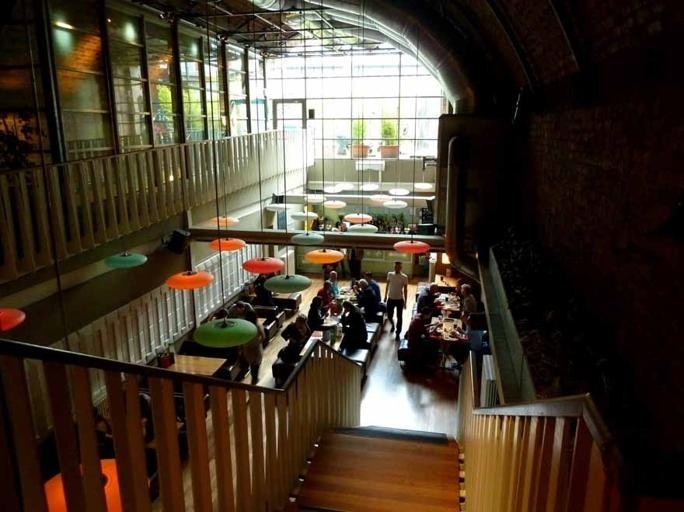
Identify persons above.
[459,284,477,321]
[383,261,408,342]
[456,310,484,352]
[409,306,433,356]
[415,283,445,317]
[455,278,464,298]
[226,269,381,386]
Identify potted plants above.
[349,119,369,159]
[379,118,399,158]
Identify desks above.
[94,416,181,452]
[144,352,224,384]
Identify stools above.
[177,425,190,456]
[271,286,388,391]
[187,280,271,383]
[399,279,489,375]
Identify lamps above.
[212,214,239,228]
[19,0,152,512]
[165,1,211,291]
[103,2,146,267]
[305,131,343,264]
[291,70,324,244]
[191,8,257,348]
[392,67,431,252]
[242,3,285,273]
[210,9,246,251]
[0,301,29,334]
[266,172,437,224]
[266,1,310,294]
